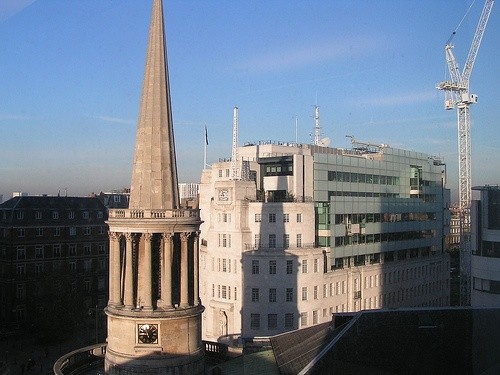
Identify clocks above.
[218,189,230,202]
[136,322,161,348]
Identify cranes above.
[434,0,497,306]
